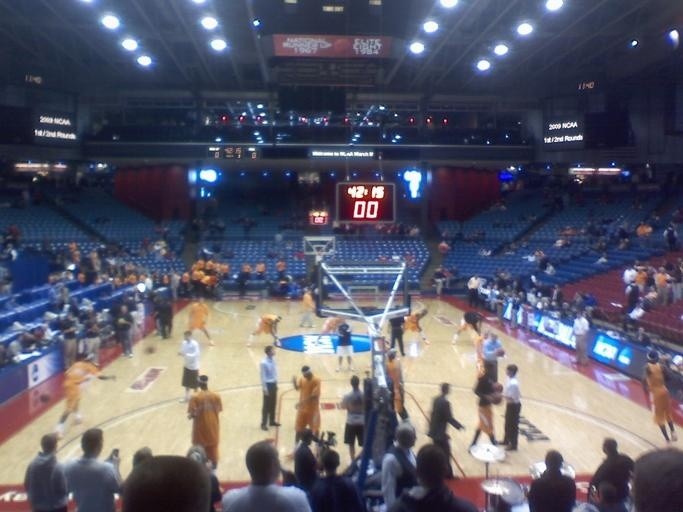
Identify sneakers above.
[335,366,356,373]
[275,340,282,347]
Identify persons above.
[2,174,682,512]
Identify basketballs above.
[496,349,504,356]
[492,393,502,404]
[438,242,449,254]
[492,382,502,393]
[40,393,50,402]
[147,345,156,353]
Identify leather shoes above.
[261,423,267,430]
[269,421,281,426]
[497,439,517,451]
[178,397,190,403]
